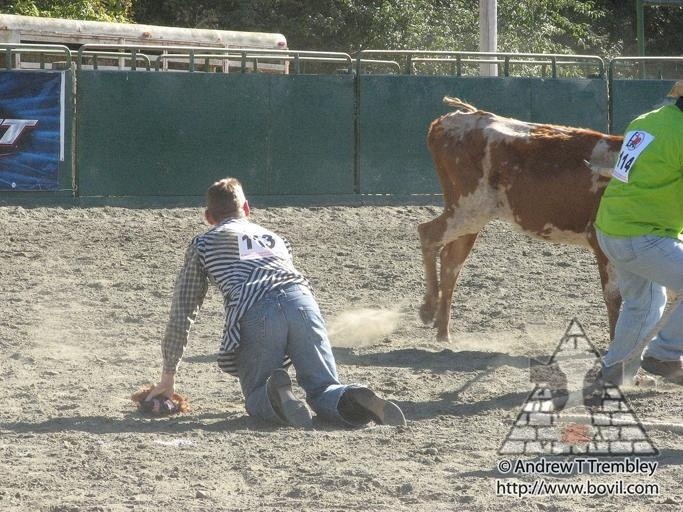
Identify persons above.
[592,92,683,391]
[130,177,408,432]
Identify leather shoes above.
[594,371,655,388]
[268,368,313,429]
[641,350,682,386]
[348,386,406,427]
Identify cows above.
[416,95,627,343]
